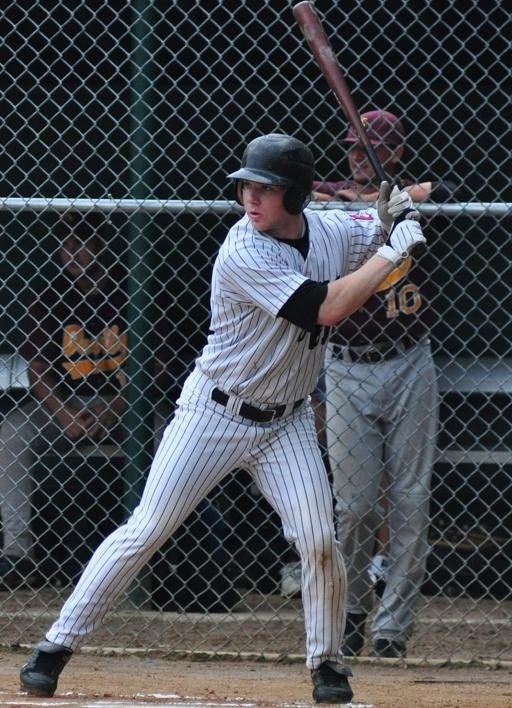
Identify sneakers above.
[368,640,406,659]
[339,614,367,657]
[282,562,303,597]
[0,554,43,591]
[20,637,73,698]
[310,660,353,705]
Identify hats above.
[339,112,406,147]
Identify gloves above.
[377,209,427,267]
[378,180,421,233]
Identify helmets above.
[224,133,315,214]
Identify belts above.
[211,388,304,422]
[332,337,420,363]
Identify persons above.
[1,209,179,590]
[301,111,484,660]
[17,131,423,706]
[277,368,395,603]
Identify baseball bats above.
[292,0,427,260]
